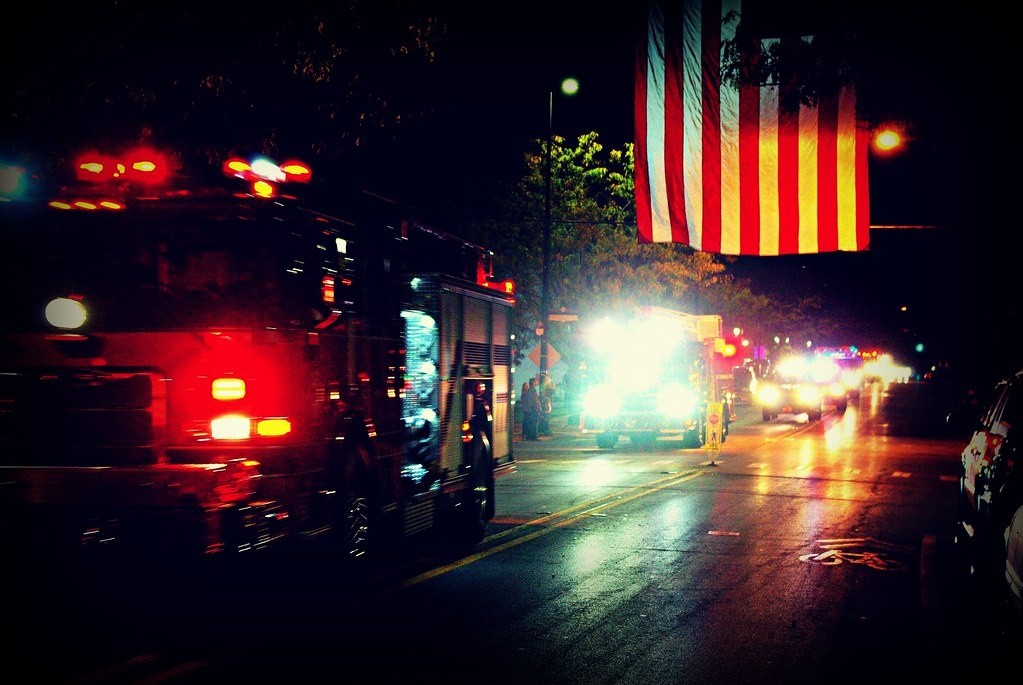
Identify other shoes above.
[519,433,528,440]
[527,436,542,441]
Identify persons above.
[521,378,556,441]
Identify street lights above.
[539,73,580,404]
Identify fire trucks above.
[0,155,519,618]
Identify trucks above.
[574,309,734,450]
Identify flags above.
[634,0,870,253]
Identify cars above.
[761,345,883,423]
